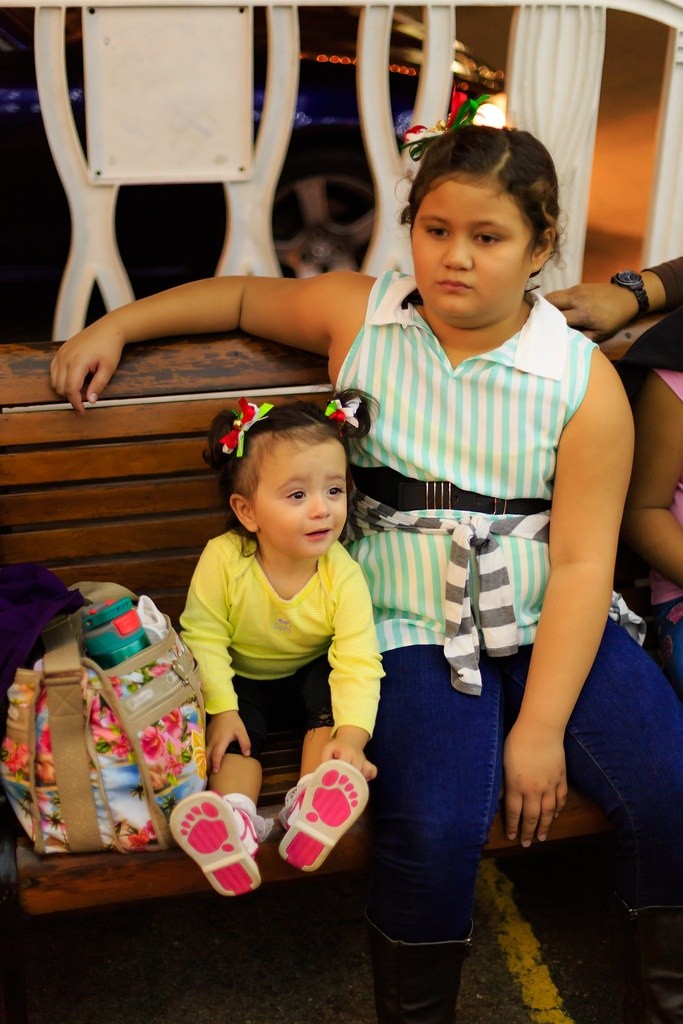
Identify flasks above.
[79,592,151,670]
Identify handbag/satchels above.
[0,581,209,853]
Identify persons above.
[620,304,683,688]
[168,387,387,897]
[47,123,683,1023]
[541,256,683,344]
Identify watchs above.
[611,271,649,320]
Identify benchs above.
[1,303,682,922]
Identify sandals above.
[278,759,370,873]
[168,788,262,898]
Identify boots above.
[364,905,474,1023]
[615,892,682,1024]
